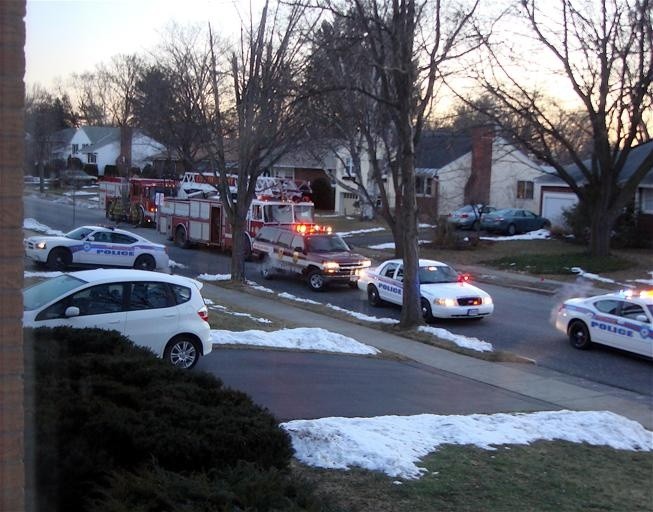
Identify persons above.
[109,194,140,229]
[213,214,220,245]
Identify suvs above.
[62,170,97,186]
[251,222,371,292]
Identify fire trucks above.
[155,172,316,262]
[98,176,178,227]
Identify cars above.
[480,208,550,236]
[24,269,213,371]
[445,204,496,232]
[22,224,169,272]
[555,288,652,360]
[357,258,495,322]
